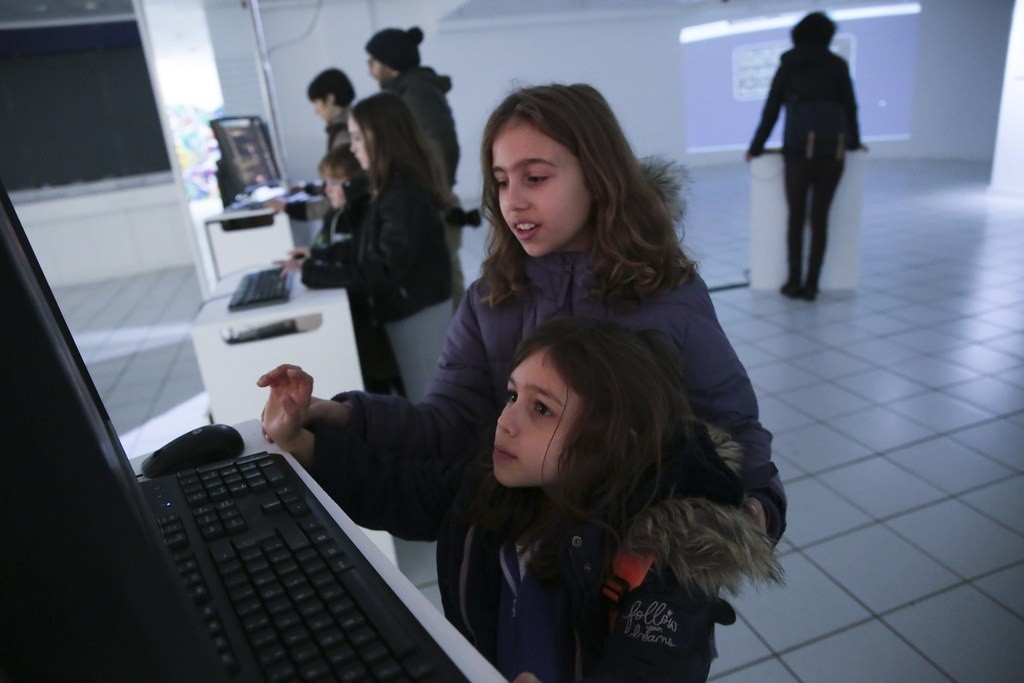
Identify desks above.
[190,263,366,426]
[203,192,323,282]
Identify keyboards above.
[247,190,304,212]
[228,266,292,311]
[134,451,473,683]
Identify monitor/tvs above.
[0,197,216,683]
[209,116,281,195]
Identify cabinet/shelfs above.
[751,146,863,293]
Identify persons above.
[265,26,481,408]
[256,318,783,682]
[260,84,787,681]
[747,11,869,301]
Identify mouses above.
[141,424,244,478]
